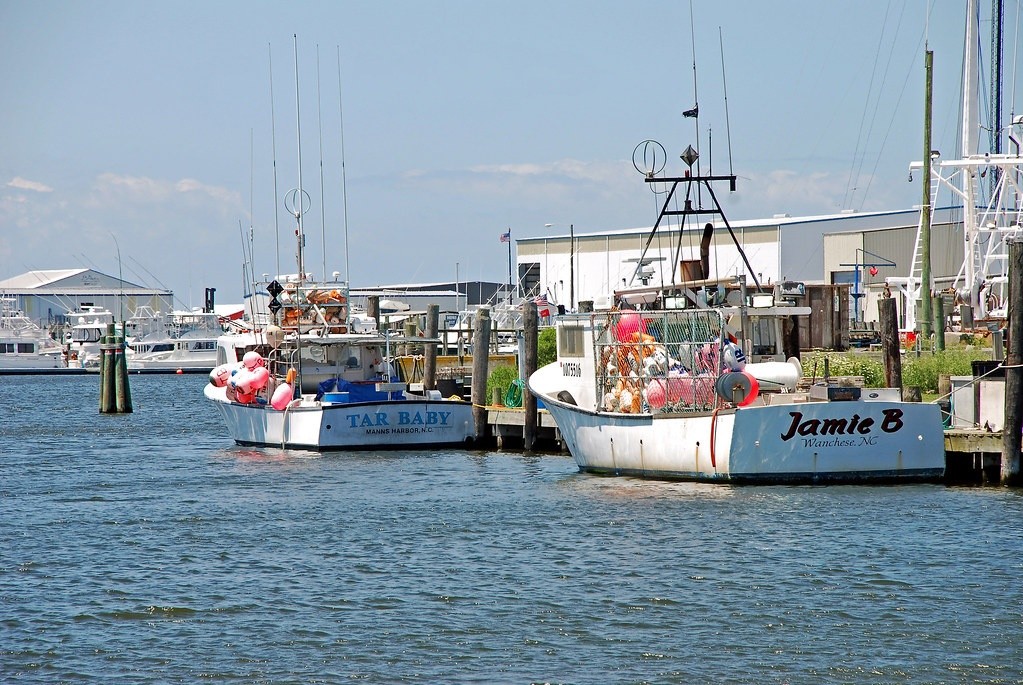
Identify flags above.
[534,294,548,306]
[540,308,550,317]
[500,233,510,242]
[682,105,698,118]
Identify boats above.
[526,139,946,484]
[202,189,476,453]
[127,313,226,372]
[0,297,86,369]
[62,306,136,368]
[436,311,503,356]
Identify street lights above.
[544,224,573,314]
